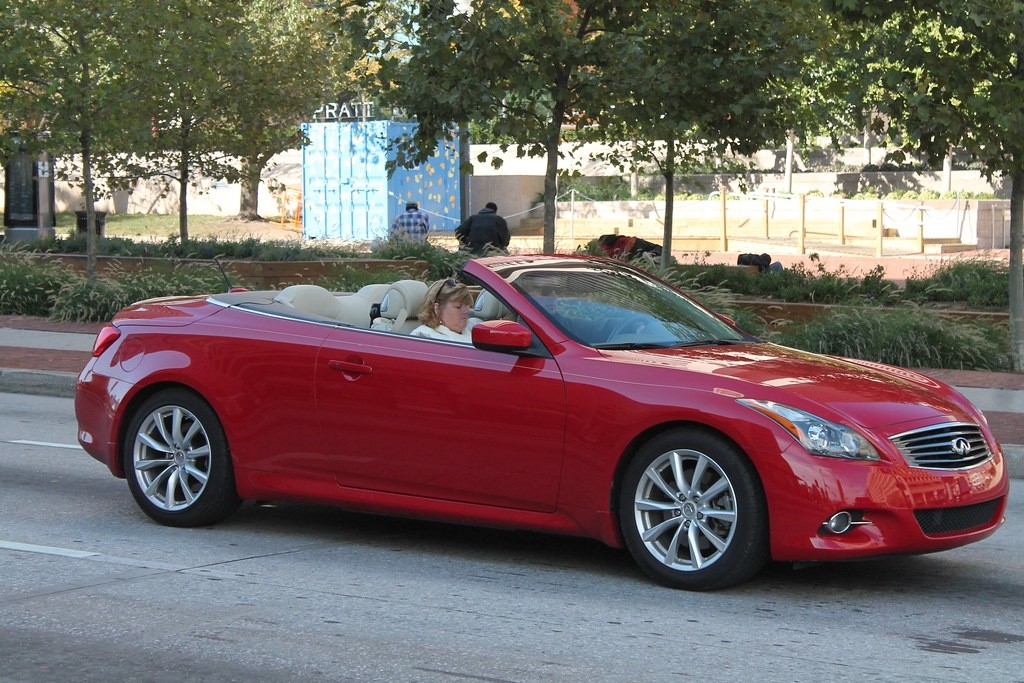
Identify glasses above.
[435,278,462,300]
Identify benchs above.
[274,284,390,329]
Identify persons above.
[511,275,637,356]
[408,279,483,344]
[391,200,429,242]
[455,203,510,255]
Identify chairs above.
[371,279,430,335]
[467,287,516,322]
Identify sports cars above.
[75,254,1010,590]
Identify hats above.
[406,202,418,208]
